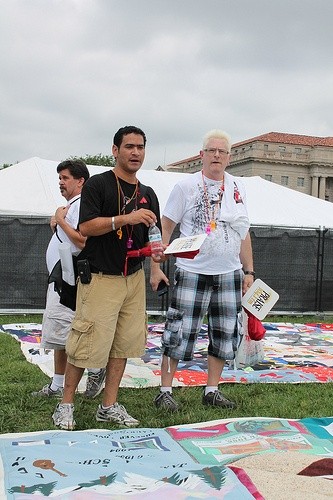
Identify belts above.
[90,262,142,276]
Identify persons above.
[150,131,255,411]
[32,161,105,398]
[53,125,164,430]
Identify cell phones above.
[156,280,167,296]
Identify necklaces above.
[110,168,139,248]
[201,169,225,232]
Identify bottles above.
[148,221,163,258]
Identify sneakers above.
[28,377,64,401]
[153,391,186,412]
[95,401,140,428]
[201,390,236,409]
[85,368,107,399]
[51,402,77,430]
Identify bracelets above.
[244,271,255,277]
[112,217,115,230]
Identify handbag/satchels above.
[235,305,265,369]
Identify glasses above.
[203,148,229,156]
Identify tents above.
[0,159,333,312]
